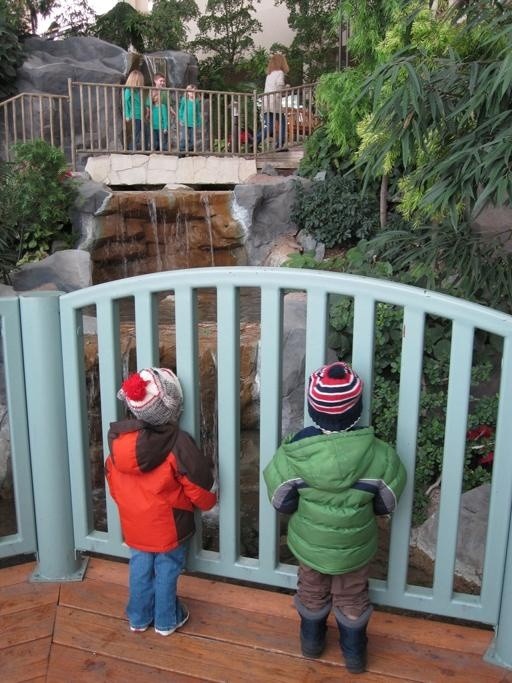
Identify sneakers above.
[130,605,189,636]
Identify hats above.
[307,363,363,434]
[117,367,183,427]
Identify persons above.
[262,358,407,674]
[151,88,169,150]
[120,67,146,151]
[103,364,219,637]
[178,82,202,158]
[145,70,175,151]
[244,52,291,152]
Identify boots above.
[333,605,373,673]
[294,593,332,658]
